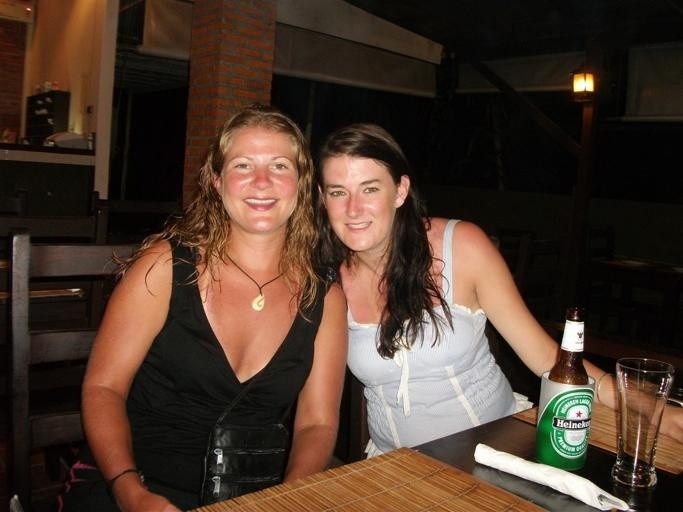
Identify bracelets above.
[596,372,615,406]
[108,469,144,488]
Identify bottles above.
[548,304,588,386]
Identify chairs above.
[9,227,146,511]
[495,233,560,354]
[589,265,655,342]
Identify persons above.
[316,121,683,458]
[57,101,349,512]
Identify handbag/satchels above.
[199,421,290,505]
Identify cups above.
[535,371,596,471]
[610,357,676,487]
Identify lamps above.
[568,66,598,102]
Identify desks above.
[184,379,683,512]
[604,256,683,344]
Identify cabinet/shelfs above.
[2,147,93,438]
[26,91,69,140]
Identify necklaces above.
[225,253,285,312]
